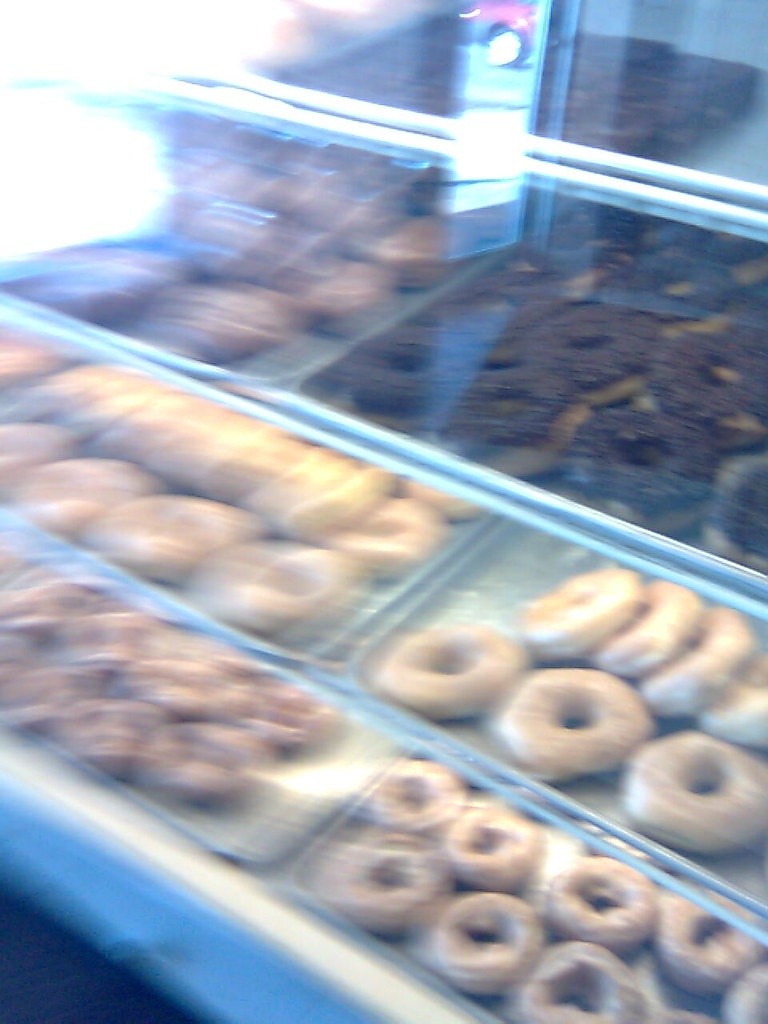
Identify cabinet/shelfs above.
[0,76,768,950]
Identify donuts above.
[0,18,768,1024]
